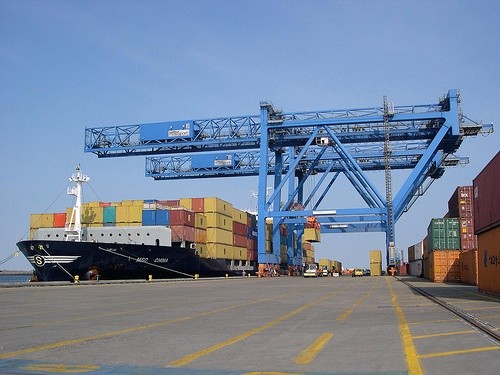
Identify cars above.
[299,262,371,279]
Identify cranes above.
[88,90,497,269]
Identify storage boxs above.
[368,250,385,279]
[401,148,500,293]
[26,197,345,276]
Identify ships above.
[15,164,246,281]
